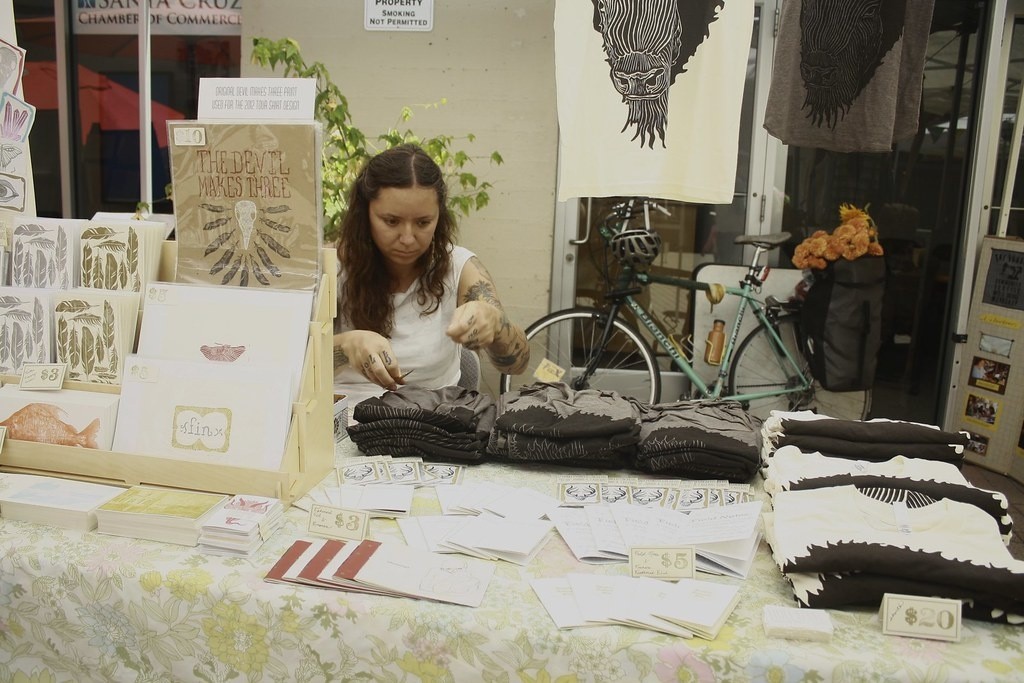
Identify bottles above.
[703,318,726,367]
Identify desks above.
[0,404,1023,681]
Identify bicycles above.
[498,195,873,431]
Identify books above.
[4,116,327,476]
[0,452,769,637]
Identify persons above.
[317,141,528,406]
